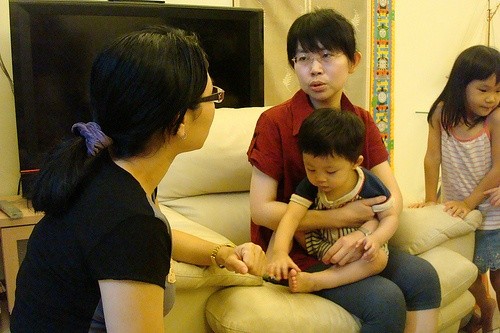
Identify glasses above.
[188,86,225,104]
[292,52,346,65]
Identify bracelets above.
[358,227,372,236]
[211,243,234,269]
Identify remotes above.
[0,200,23,219]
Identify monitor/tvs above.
[8,0,264,198]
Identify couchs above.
[160,106,482,332]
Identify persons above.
[247,9,441,333]
[408,45,500,333]
[265,107,398,292]
[10,26,267,333]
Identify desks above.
[0,194,45,316]
